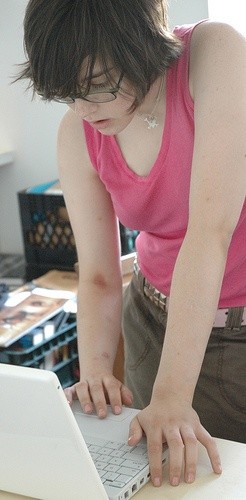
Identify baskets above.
[0,316,79,389]
[16,189,139,263]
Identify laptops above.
[0,363,172,500]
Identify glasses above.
[49,68,125,104]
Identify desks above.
[0,438,246,500]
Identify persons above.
[9,0,246,487]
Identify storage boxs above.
[18,190,138,283]
[0,314,80,389]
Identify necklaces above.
[135,73,164,130]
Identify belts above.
[133,257,246,328]
